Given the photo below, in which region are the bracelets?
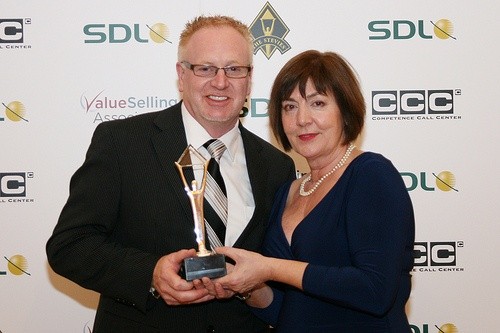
[233,289,254,301]
[149,286,162,299]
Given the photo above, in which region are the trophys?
[174,142,227,281]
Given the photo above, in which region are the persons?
[45,14,299,333]
[189,50,415,333]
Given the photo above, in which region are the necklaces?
[299,142,357,197]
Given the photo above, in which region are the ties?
[203,139,229,252]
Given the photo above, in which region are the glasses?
[182,59,253,78]
